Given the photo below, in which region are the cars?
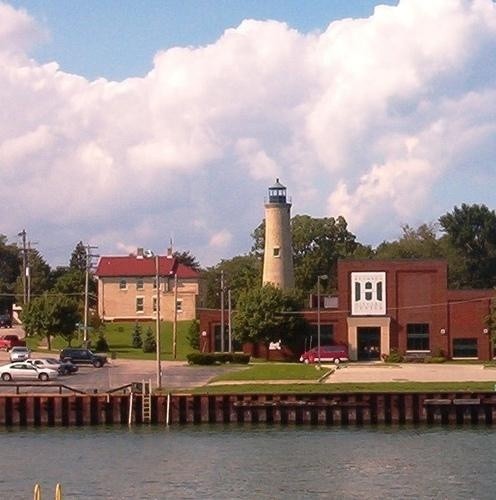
[0,333,81,383]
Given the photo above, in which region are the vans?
[298,345,350,364]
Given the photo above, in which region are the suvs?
[0,313,12,329]
[59,346,108,368]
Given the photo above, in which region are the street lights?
[26,240,40,305]
[170,270,177,359]
[144,248,163,390]
[222,284,233,354]
[17,227,26,304]
[316,274,329,370]
[215,287,225,353]
